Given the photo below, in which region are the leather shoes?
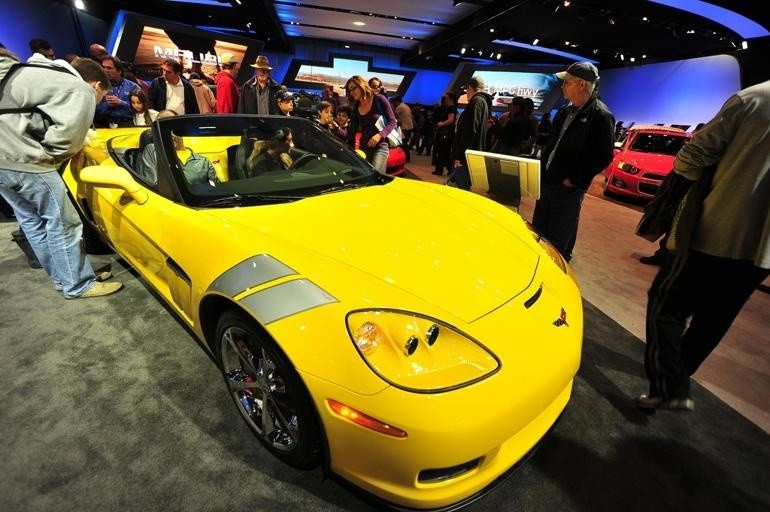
[637,388,694,413]
[640,253,668,266]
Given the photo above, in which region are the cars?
[604,125,695,205]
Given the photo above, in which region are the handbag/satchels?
[437,126,452,142]
[375,114,404,148]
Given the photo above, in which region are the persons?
[0,51,126,300]
[633,79,770,414]
[531,61,618,265]
[1,36,707,269]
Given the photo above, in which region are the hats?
[459,79,481,89]
[250,55,273,70]
[556,62,599,82]
[274,90,296,100]
[219,53,238,64]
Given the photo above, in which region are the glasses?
[370,84,382,88]
[349,86,357,92]
[564,79,582,85]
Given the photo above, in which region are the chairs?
[135,129,154,178]
[236,132,259,182]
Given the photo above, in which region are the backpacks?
[0,47,76,116]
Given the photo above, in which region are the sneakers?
[65,282,122,299]
[56,272,111,292]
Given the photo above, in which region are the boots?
[11,229,43,269]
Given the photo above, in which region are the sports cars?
[56,114,585,512]
